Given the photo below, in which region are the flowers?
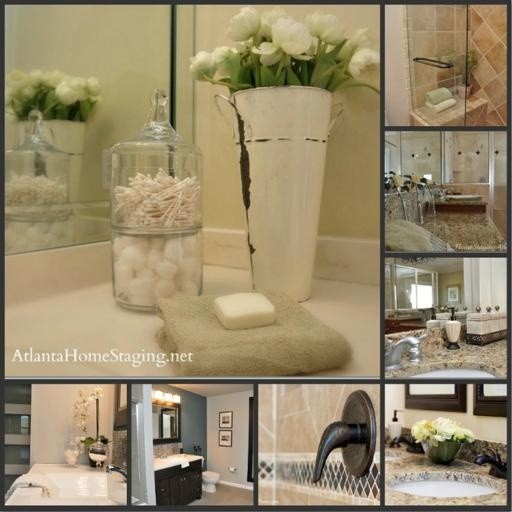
[190,6,380,88]
[384,171,410,193]
[73,386,112,449]
[412,418,477,447]
[402,173,427,195]
[2,68,101,124]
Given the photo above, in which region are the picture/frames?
[219,411,233,428]
[446,283,461,306]
[219,430,232,447]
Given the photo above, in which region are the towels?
[425,87,452,104]
[175,459,190,469]
[425,99,457,112]
[154,289,352,377]
[4,473,61,502]
[385,219,455,252]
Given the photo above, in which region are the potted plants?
[443,48,479,98]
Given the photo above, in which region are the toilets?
[200,470,221,494]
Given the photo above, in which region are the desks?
[425,200,488,214]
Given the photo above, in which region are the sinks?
[393,478,497,498]
[45,470,109,499]
[410,366,498,379]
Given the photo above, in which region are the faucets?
[472,448,507,480]
[389,435,425,455]
[386,335,421,371]
[105,462,127,483]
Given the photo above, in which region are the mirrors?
[0,1,199,259]
[405,384,467,412]
[152,403,181,445]
[113,383,127,431]
[473,384,508,417]
[385,258,474,336]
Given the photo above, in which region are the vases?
[88,446,108,468]
[215,88,345,303]
[26,118,86,203]
[421,439,463,465]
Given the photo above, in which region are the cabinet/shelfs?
[155,460,203,507]
